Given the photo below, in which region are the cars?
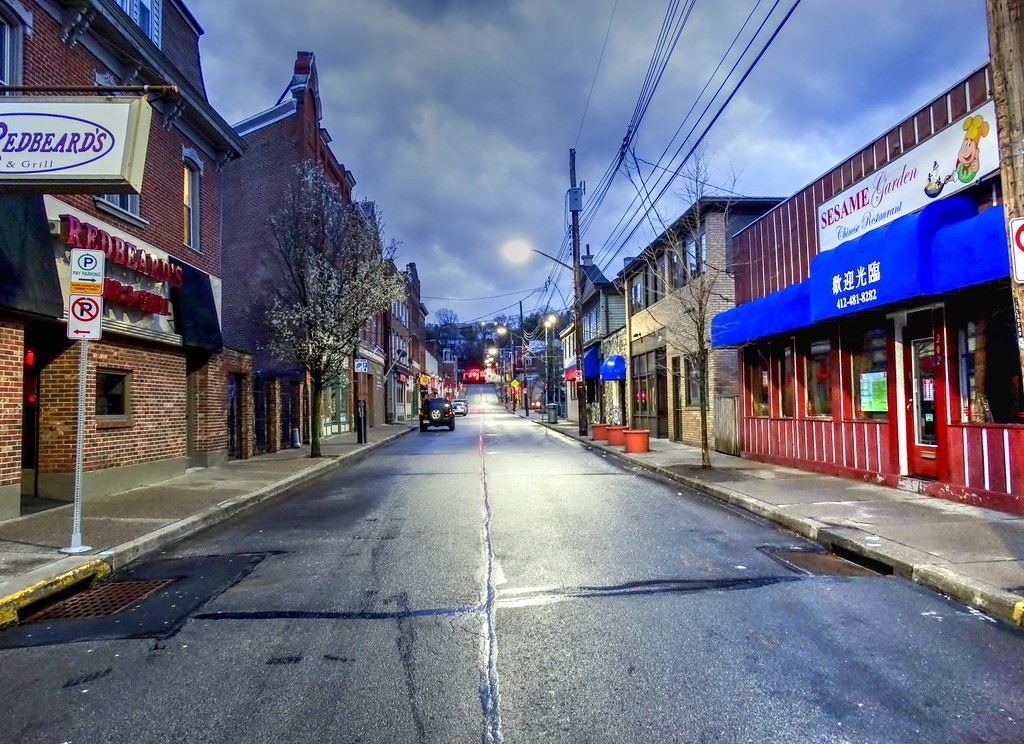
[532,395,564,410]
[451,402,467,416]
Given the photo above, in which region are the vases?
[622,429,650,454]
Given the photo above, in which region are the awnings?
[599,355,626,380]
[711,196,1010,350]
[564,347,600,381]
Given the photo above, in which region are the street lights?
[489,348,508,410]
[497,327,529,417]
[544,314,557,406]
[501,240,589,436]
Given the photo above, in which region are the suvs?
[419,397,457,432]
[455,399,469,412]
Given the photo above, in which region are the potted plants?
[586,383,630,446]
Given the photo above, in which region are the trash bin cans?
[548,403,558,423]
